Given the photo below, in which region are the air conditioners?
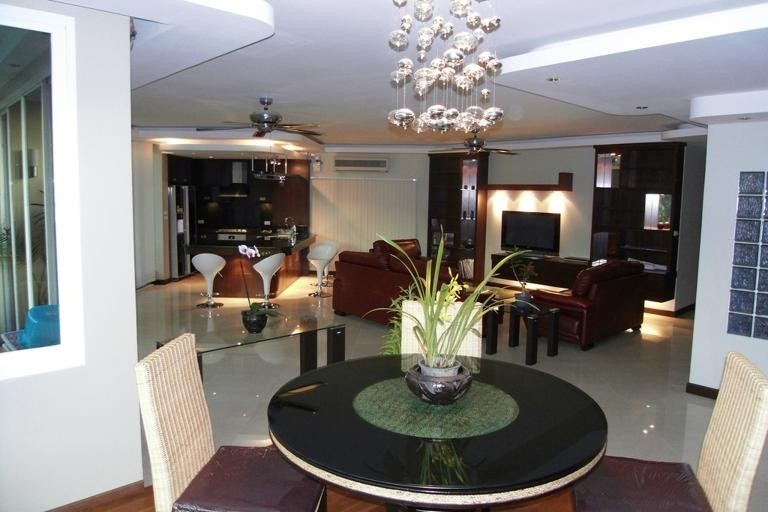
[332,156,390,174]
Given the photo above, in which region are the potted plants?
[240,300,286,334]
[357,224,544,403]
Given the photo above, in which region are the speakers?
[333,158,388,170]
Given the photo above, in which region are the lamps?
[385,1,504,136]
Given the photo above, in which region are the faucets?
[284,217,295,228]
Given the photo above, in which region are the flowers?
[505,244,539,294]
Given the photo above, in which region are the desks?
[483,296,560,365]
[155,311,346,386]
[266,347,611,510]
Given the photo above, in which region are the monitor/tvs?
[502,211,560,259]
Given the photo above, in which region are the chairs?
[303,238,340,297]
[192,252,228,309]
[568,349,768,511]
[400,299,483,361]
[252,250,287,308]
[136,329,330,511]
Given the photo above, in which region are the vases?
[514,291,532,308]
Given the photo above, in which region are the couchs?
[329,236,492,329]
[522,255,648,350]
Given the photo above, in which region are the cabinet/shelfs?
[589,141,687,302]
[426,152,490,286]
[491,250,592,288]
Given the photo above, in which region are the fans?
[194,96,327,145]
[428,131,519,158]
